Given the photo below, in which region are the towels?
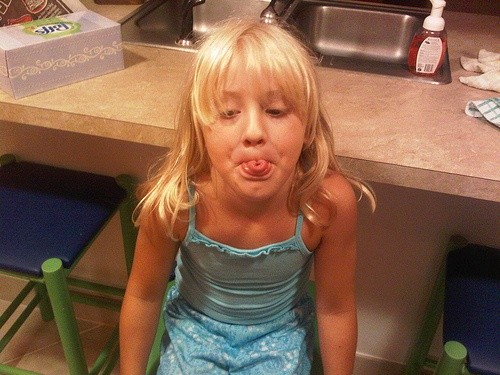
[458,48,500,93]
[464,97,500,128]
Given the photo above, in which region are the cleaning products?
[407,0,447,77]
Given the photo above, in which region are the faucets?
[176,0,205,47]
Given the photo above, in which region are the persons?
[117,15,377,375]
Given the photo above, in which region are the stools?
[0,153,142,375]
[145,278,324,375]
[401,234,500,375]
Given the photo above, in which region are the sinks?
[116,0,283,54]
[279,0,452,85]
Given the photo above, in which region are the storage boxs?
[0,9,124,100]
